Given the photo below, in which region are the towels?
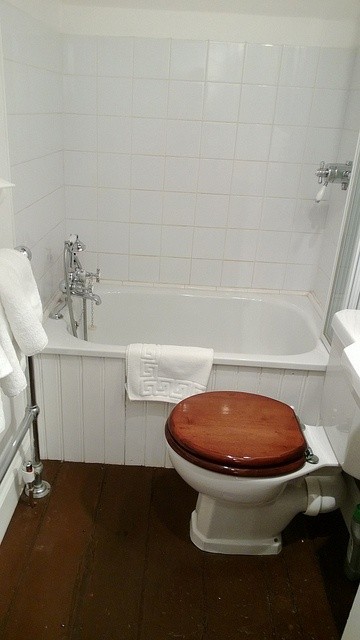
[0,247,48,397]
[125,342,213,405]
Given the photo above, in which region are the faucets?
[71,280,102,306]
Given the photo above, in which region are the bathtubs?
[28,276,344,471]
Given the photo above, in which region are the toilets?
[164,309,360,557]
